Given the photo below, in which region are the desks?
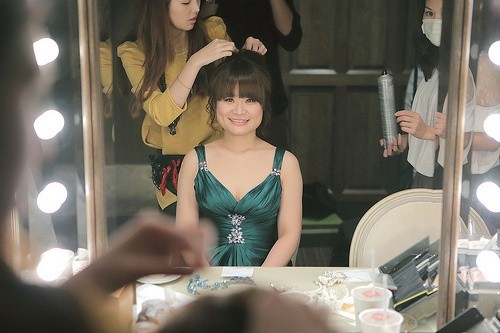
[130,267,387,333]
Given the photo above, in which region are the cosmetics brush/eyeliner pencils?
[413,248,440,280]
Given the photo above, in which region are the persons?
[118,0,268,219]
[39,0,115,254]
[190,0,309,151]
[379,0,499,242]
[0,0,116,333]
[172,48,303,267]
[155,286,353,333]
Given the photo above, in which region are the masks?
[421,19,442,48]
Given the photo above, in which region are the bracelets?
[206,1,217,4]
[176,76,191,89]
[176,77,193,91]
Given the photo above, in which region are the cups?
[73,257,90,275]
[352,286,392,333]
[359,309,403,333]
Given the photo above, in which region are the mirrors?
[77,0,473,333]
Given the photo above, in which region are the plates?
[132,284,195,322]
[137,274,181,284]
